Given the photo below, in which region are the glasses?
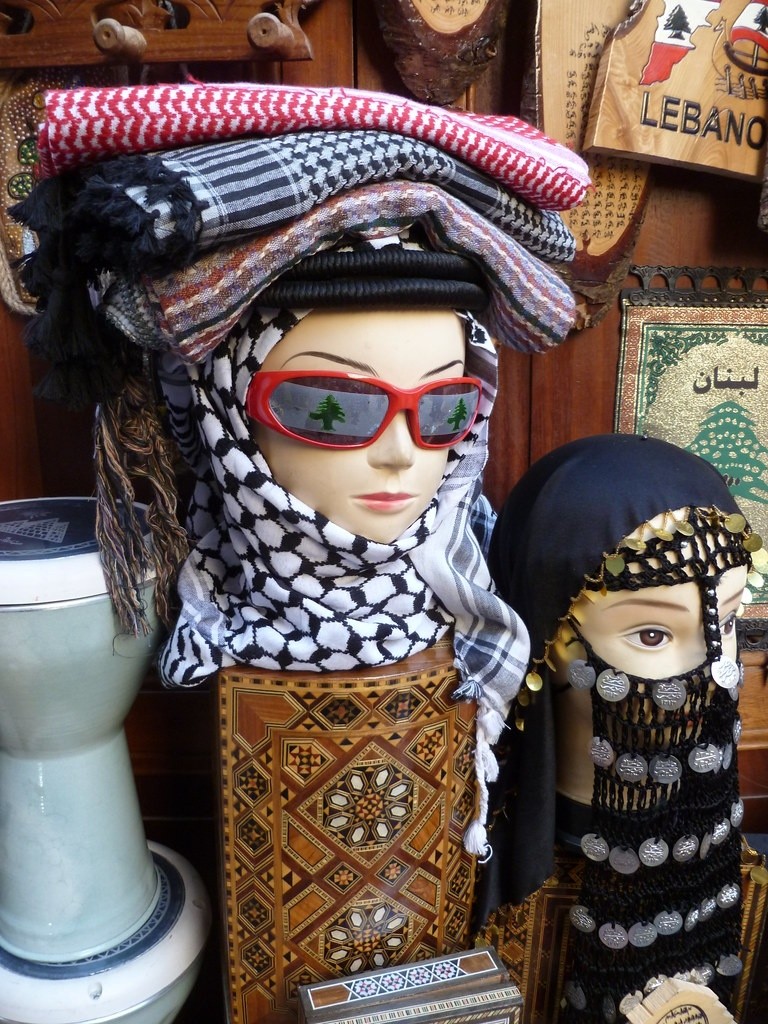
[246,370,483,451]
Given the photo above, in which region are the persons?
[160,222,493,670]
[470,434,768,812]
